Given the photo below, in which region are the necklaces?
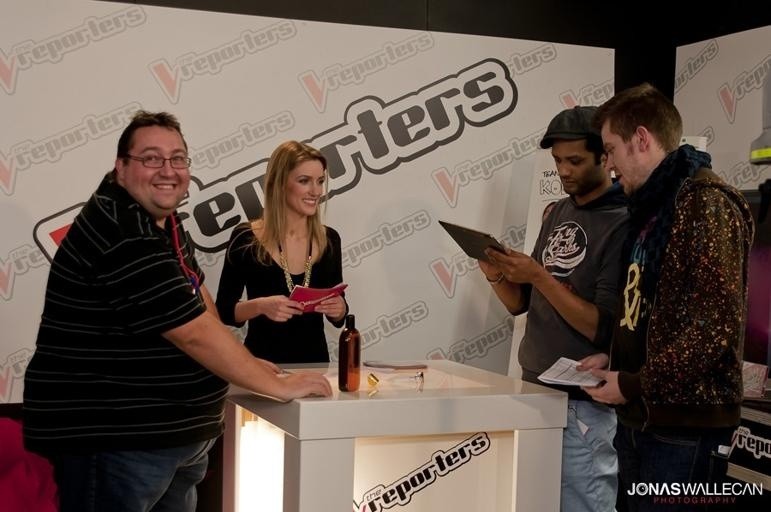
[275,234,313,293]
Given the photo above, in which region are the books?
[289,282,348,313]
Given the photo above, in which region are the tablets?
[438,219,510,261]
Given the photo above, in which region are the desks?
[220,358,570,511]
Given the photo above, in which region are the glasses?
[124,154,192,169]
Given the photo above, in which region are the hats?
[539,106,600,148]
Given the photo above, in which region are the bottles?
[338,314,362,392]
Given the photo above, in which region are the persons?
[479,106,630,510]
[215,141,349,367]
[23,113,331,512]
[573,83,755,511]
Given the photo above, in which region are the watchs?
[487,275,505,285]
